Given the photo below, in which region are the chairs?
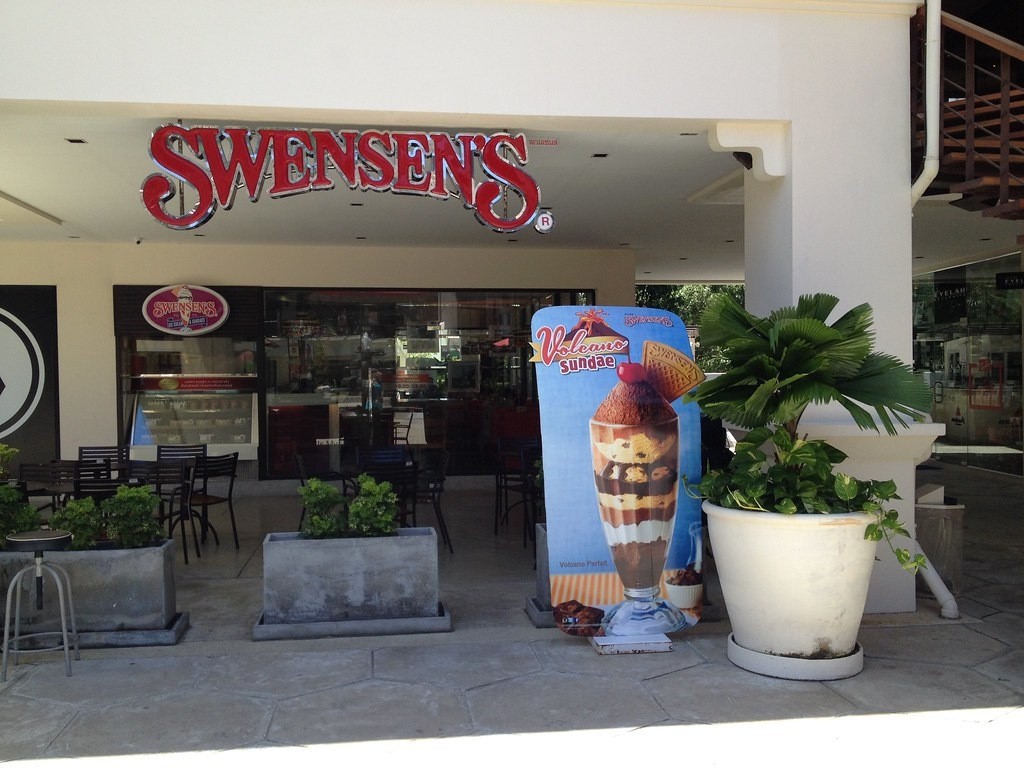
[19,445,239,565]
[494,436,546,571]
[293,411,454,554]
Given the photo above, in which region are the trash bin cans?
[916,502,966,602]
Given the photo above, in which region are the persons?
[365,372,384,411]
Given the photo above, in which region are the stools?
[1,531,80,682]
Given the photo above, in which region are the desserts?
[591,378,678,589]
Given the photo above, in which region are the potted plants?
[0,443,190,649]
[252,472,452,642]
[684,293,934,680]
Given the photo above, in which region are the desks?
[45,478,147,494]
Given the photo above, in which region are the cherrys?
[617,345,644,383]
